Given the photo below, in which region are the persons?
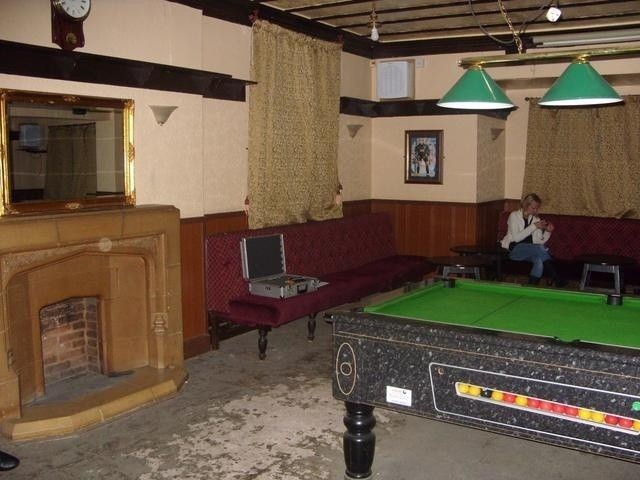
[0,450,21,474]
[501,192,557,286]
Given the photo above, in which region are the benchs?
[205,211,436,361]
[496,210,640,274]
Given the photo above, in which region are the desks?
[330,272,640,479]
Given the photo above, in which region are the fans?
[377,61,416,100]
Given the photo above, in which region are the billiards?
[458,383,640,432]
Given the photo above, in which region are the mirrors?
[0,88,134,217]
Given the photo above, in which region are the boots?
[543,259,568,288]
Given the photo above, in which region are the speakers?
[19,124,42,147]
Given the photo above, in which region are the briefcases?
[240,234,317,297]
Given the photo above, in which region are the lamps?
[435,46,639,110]
[370,2,380,42]
[150,105,177,126]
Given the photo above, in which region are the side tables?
[576,255,635,296]
[425,245,507,280]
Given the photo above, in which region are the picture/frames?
[405,131,442,184]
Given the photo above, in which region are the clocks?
[50,0,91,51]
